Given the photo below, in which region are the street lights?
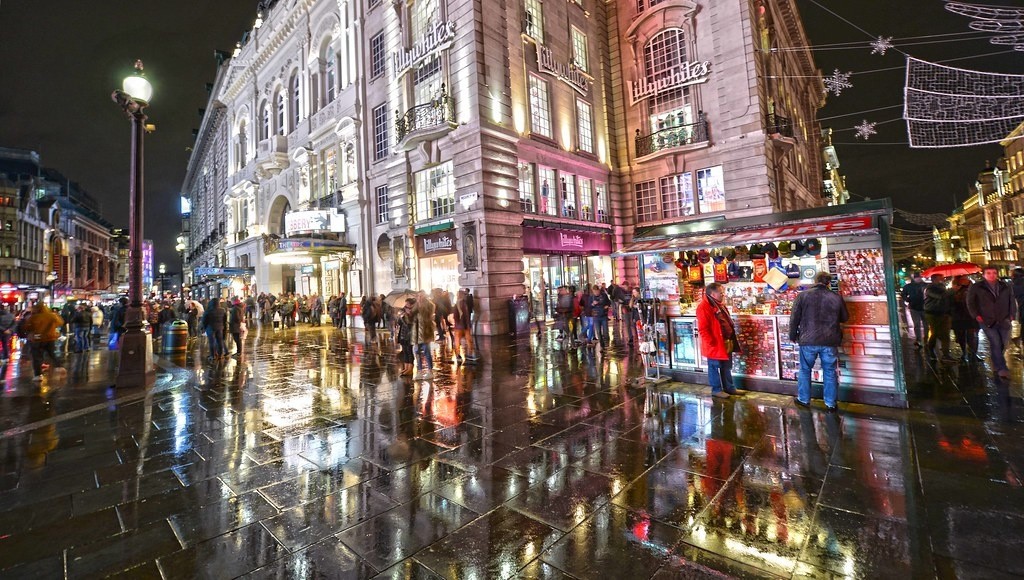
[45,275,55,296]
[110,57,156,389]
[159,263,166,301]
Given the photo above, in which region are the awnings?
[609,214,881,261]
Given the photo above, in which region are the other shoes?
[412,373,423,380]
[32,375,43,381]
[557,336,563,340]
[224,352,229,356]
[794,398,810,408]
[403,370,412,375]
[423,373,433,379]
[713,391,730,399]
[467,355,475,360]
[930,351,936,360]
[401,369,407,374]
[827,405,838,414]
[457,355,461,361]
[730,390,746,395]
[998,369,1009,376]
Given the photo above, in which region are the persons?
[901,271,929,349]
[506,279,640,350]
[788,273,850,416]
[925,266,1024,364]
[696,283,746,399]
[358,289,480,381]
[966,267,1016,377]
[0,285,348,394]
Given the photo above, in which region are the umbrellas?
[919,263,983,278]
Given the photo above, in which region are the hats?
[914,273,919,278]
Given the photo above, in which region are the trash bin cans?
[507,295,531,336]
